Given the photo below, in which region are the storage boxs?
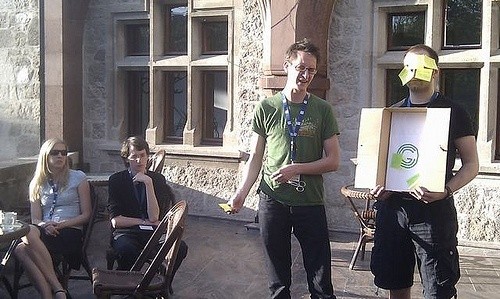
[354,107,451,192]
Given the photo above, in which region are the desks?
[341,182,379,270]
[84,172,110,187]
[0,213,30,299]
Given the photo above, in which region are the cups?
[0,209,18,231]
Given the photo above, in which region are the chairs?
[92,199,190,299]
[106,183,176,270]
[12,191,98,299]
[147,148,166,176]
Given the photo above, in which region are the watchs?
[445,186,453,200]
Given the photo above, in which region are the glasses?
[289,60,318,76]
[49,150,67,156]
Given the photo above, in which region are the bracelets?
[142,218,146,225]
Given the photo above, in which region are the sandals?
[54,289,71,299]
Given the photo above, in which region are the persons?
[107,136,188,287]
[225,39,341,299]
[14,136,92,299]
[371,44,480,299]
